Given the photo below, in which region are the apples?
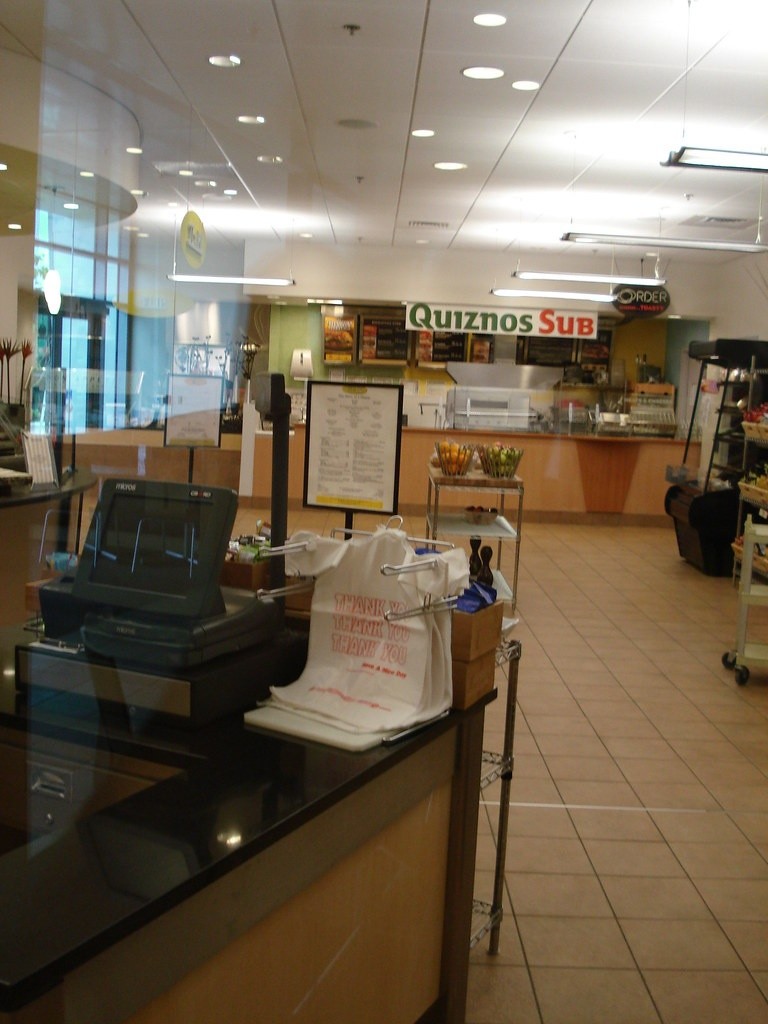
[478,442,519,476]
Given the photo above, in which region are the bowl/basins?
[463,510,499,525]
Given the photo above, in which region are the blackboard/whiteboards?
[359,316,412,365]
[577,327,613,385]
[516,334,578,366]
[415,329,471,368]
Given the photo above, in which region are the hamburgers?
[324,331,352,350]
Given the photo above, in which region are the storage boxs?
[450,648,497,709]
[451,600,505,660]
[221,560,314,611]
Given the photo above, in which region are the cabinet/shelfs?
[666,339,768,686]
[424,462,525,613]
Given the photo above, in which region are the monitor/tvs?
[70,477,238,617]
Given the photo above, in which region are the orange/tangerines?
[438,441,470,474]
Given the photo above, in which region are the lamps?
[658,0,768,174]
[166,208,296,287]
[487,156,768,303]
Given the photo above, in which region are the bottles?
[639,354,648,382]
[635,354,642,379]
